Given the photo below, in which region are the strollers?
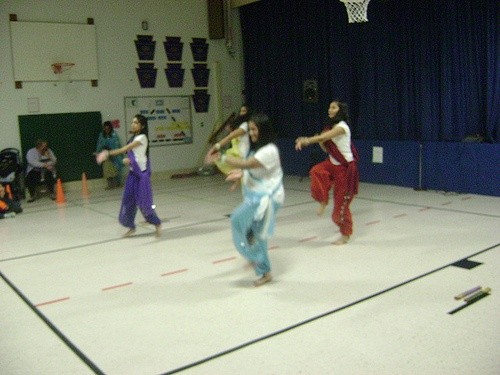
[0,148,25,214]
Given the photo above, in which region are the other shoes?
[27,192,41,202]
[48,190,56,200]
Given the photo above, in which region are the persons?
[24,138,57,203]
[97,114,162,237]
[295,100,359,245]
[95,121,123,190]
[206,102,254,192]
[215,113,285,285]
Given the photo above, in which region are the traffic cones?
[54,179,66,204]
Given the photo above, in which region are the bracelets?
[304,137,308,146]
[106,150,111,158]
[215,142,221,151]
[221,154,228,164]
